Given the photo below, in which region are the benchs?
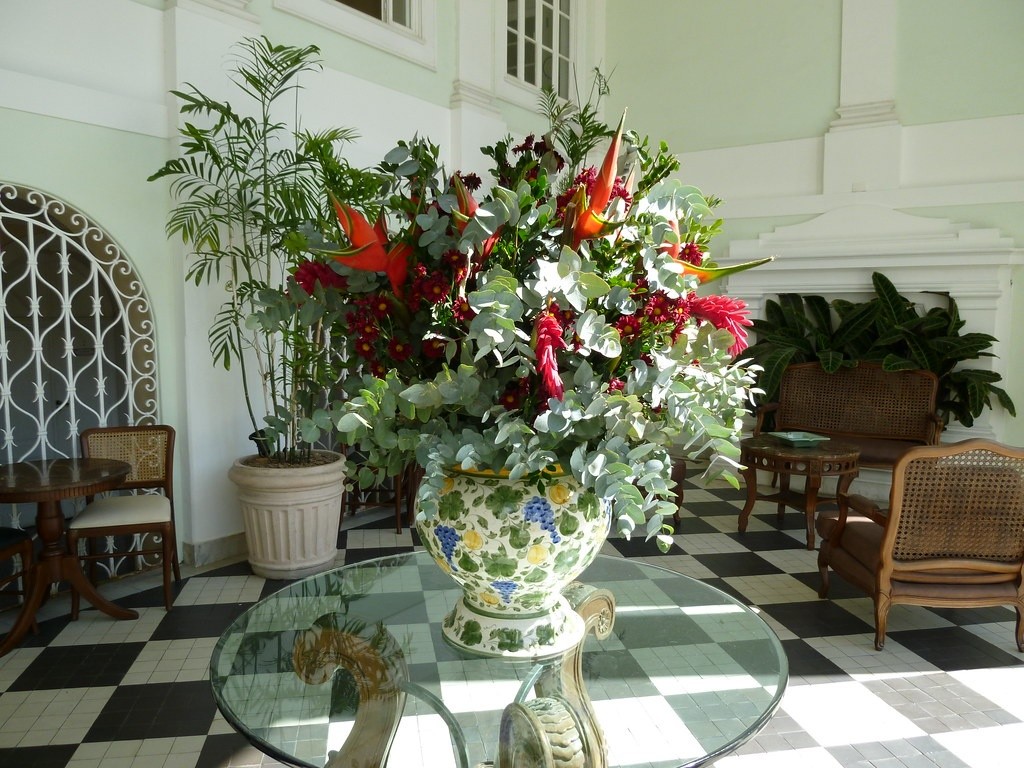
[754,361,944,487]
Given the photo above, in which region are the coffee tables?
[737,434,862,551]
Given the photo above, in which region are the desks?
[0,458,139,657]
[209,551,789,768]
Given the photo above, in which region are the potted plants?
[145,36,405,580]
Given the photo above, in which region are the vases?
[413,465,615,660]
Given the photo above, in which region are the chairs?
[66,425,181,621]
[816,438,1024,652]
[340,441,415,534]
[0,527,38,634]
[632,458,686,531]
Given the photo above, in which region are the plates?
[767,431,831,448]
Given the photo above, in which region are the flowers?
[246,53,766,537]
